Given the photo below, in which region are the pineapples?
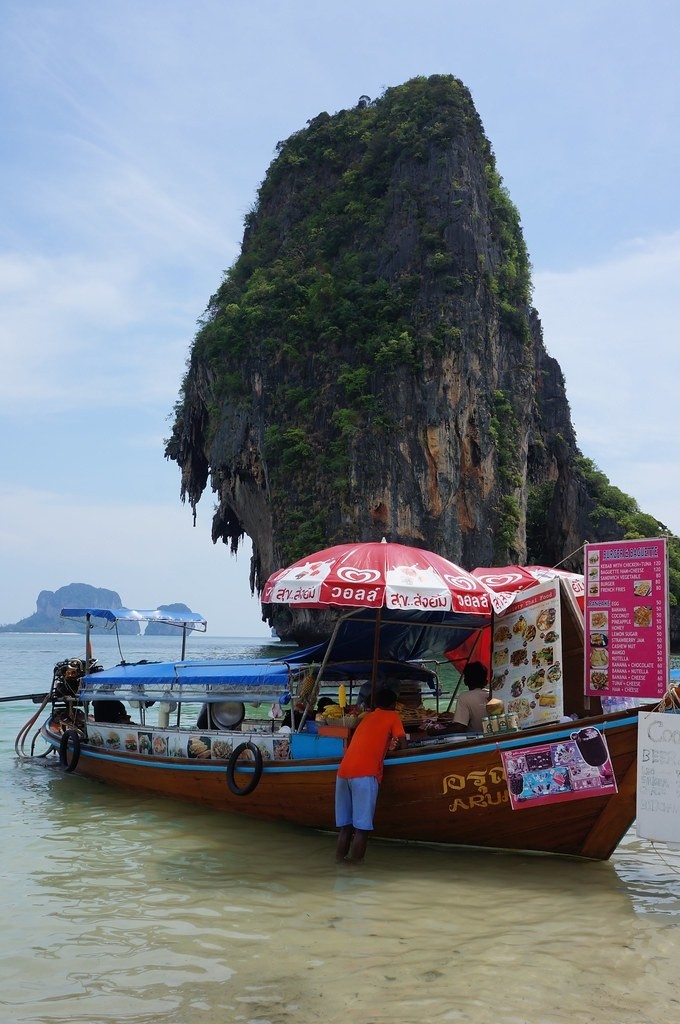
[302,666,315,694]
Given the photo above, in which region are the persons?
[92,683,137,725]
[196,683,223,730]
[426,660,491,739]
[278,679,374,734]
[335,689,410,859]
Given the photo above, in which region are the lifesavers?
[59,730,80,772]
[227,741,263,796]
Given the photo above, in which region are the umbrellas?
[441,562,585,684]
[261,537,516,720]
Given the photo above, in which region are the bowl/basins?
[535,609,555,632]
[526,675,544,692]
[546,665,561,684]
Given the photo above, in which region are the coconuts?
[486,698,504,716]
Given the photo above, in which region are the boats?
[38,657,680,860]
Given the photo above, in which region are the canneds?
[480,711,518,733]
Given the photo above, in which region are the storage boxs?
[305,721,327,734]
[318,725,351,738]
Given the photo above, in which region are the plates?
[506,696,534,720]
[590,633,608,647]
[494,624,508,645]
[589,611,608,631]
[512,621,527,638]
[590,648,609,670]
[522,625,536,643]
[530,646,553,667]
[510,648,528,668]
[509,677,525,698]
[492,650,510,670]
[543,629,560,646]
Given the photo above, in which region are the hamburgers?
[106,732,120,749]
[125,733,137,752]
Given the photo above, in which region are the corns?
[322,705,342,719]
[358,712,368,718]
[190,743,211,759]
[339,684,346,707]
